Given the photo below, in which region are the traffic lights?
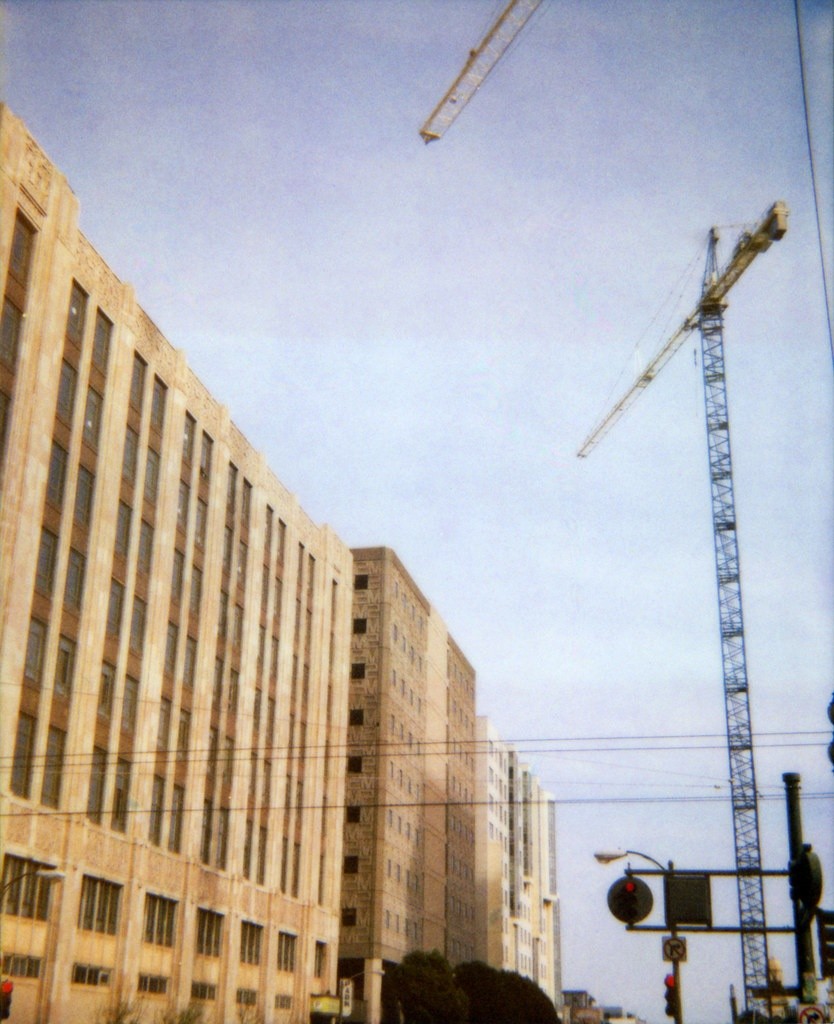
[664,974,677,1017]
[622,878,635,924]
[818,909,834,978]
[1,980,15,1017]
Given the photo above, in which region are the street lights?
[340,969,384,1024]
[591,847,682,1024]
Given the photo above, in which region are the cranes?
[573,194,794,1023]
